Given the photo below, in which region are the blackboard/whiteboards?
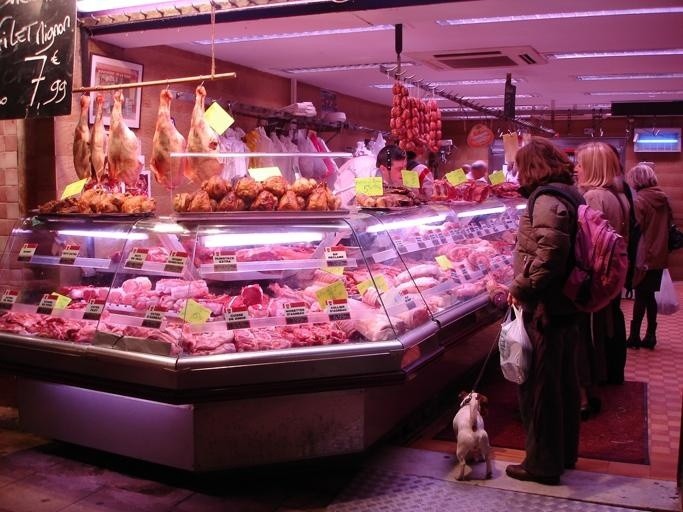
[0,0,77,120]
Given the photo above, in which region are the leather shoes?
[507,464,561,485]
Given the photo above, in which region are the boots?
[626,320,642,350]
[642,319,657,350]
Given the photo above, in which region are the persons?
[577,141,673,419]
[493,135,587,486]
[335,145,488,208]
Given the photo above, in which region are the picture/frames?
[89,55,144,132]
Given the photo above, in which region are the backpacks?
[529,185,630,313]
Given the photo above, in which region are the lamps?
[636,139,680,145]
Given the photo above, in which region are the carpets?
[432,372,650,464]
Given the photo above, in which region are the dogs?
[452,391,492,480]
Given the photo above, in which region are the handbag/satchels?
[668,225,683,250]
